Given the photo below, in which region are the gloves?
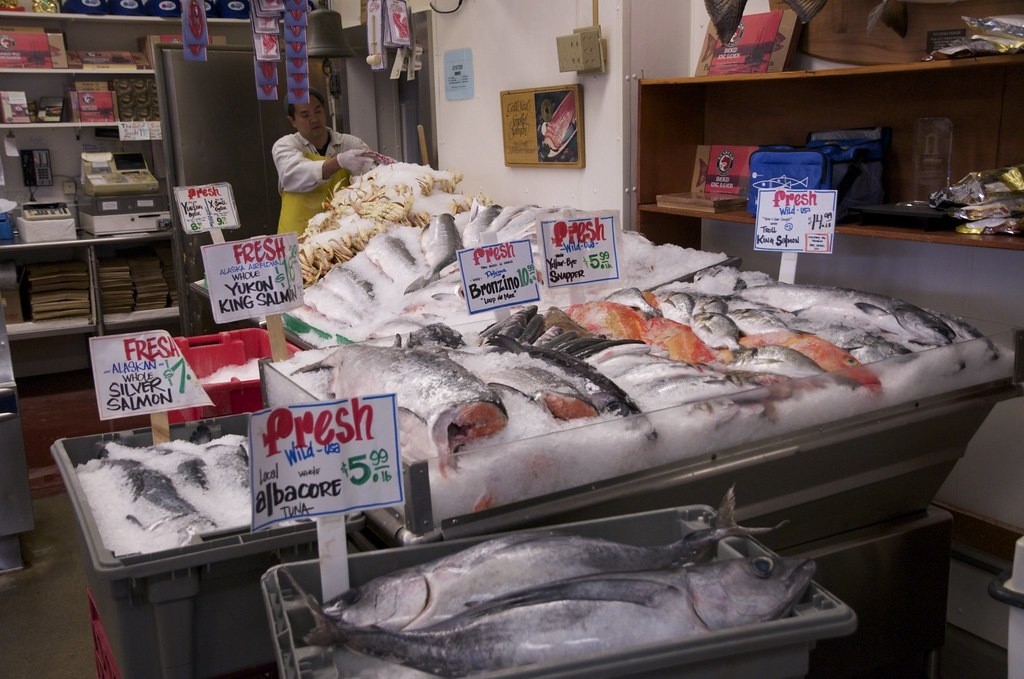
[337,149,373,170]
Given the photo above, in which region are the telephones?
[19,149,53,187]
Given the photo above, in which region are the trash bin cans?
[988,536,1024,679]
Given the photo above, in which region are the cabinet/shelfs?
[636,55,1024,255]
[0,19,259,337]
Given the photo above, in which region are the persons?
[272,88,378,239]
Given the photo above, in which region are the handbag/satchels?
[749,126,891,223]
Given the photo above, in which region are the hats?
[283,87,325,113]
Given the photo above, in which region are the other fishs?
[289,265,1001,510]
[83,433,250,544]
[278,554,821,679]
[297,164,495,289]
[293,205,584,339]
[322,487,790,631]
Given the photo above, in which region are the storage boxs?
[51,414,366,678]
[0,26,153,70]
[0,79,120,124]
[259,503,859,678]
[0,266,28,323]
[167,329,302,424]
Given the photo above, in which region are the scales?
[20,201,72,221]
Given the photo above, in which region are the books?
[30,248,180,322]
